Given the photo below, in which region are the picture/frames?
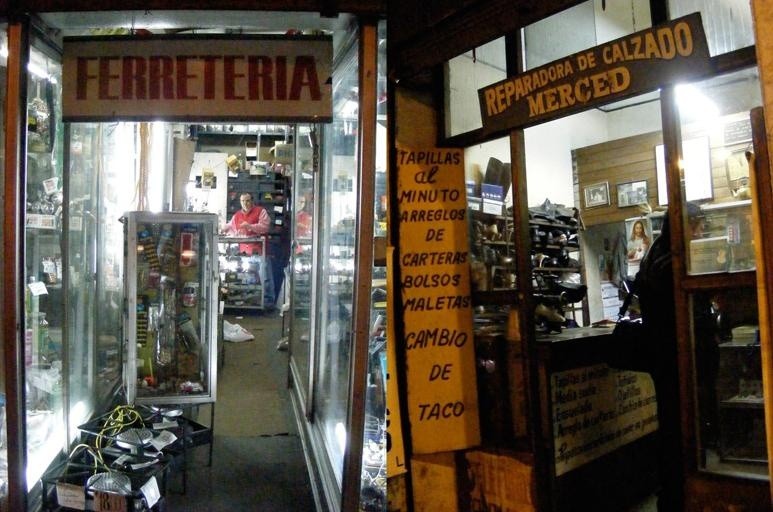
[615,179,649,208]
[583,180,611,210]
[723,117,753,147]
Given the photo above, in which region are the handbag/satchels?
[608,320,652,372]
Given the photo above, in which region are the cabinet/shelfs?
[218,169,287,316]
[40,405,186,511]
[470,209,590,328]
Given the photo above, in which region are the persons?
[628,187,646,204]
[296,191,312,237]
[221,191,276,316]
[636,200,721,512]
[627,221,649,261]
